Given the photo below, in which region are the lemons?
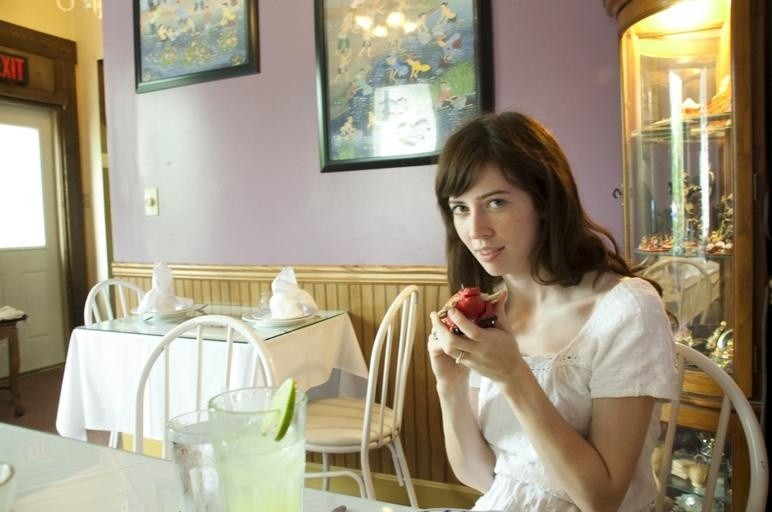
[260,378,296,443]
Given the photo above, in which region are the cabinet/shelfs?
[598,0,772,512]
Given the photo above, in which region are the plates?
[129,296,194,320]
[241,305,314,327]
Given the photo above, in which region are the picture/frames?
[314,0,496,173]
[132,0,262,94]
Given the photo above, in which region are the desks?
[0,305,31,420]
[75,305,347,483]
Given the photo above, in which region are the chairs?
[284,284,422,511]
[83,279,182,455]
[135,314,280,511]
[640,339,769,512]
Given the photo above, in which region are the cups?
[168,408,224,511]
[209,385,307,511]
[687,465,707,488]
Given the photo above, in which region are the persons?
[332,3,456,138]
[148,0,238,42]
[427,112,679,512]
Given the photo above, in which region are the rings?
[454,351,465,364]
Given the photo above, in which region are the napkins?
[270,265,318,320]
[135,260,182,314]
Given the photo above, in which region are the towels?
[266,262,320,322]
[127,259,186,319]
